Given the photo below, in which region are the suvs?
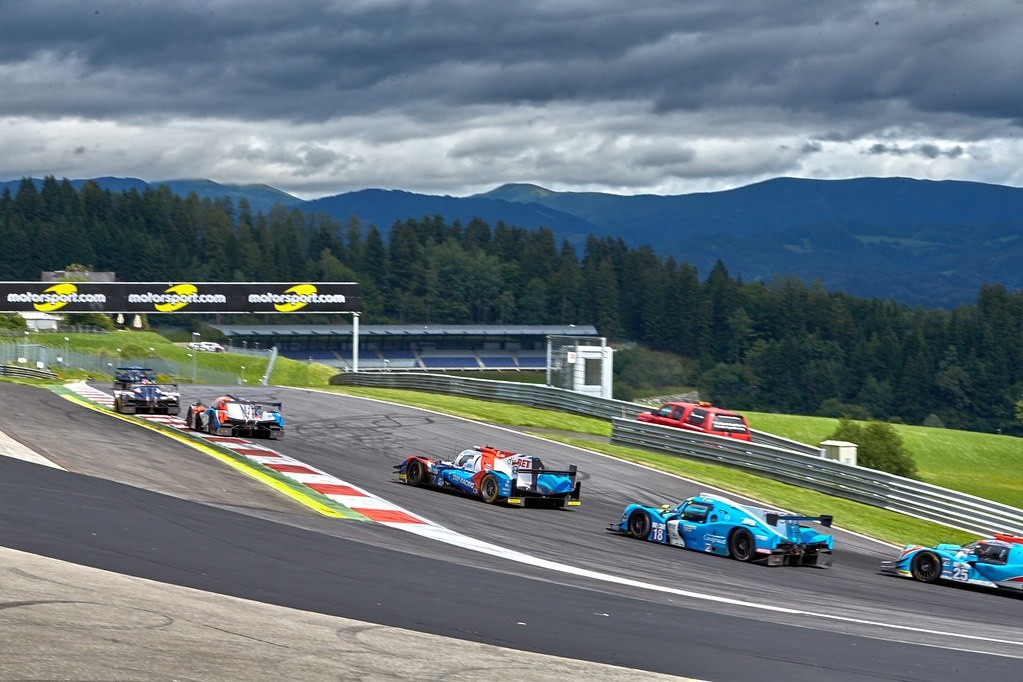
[637,401,752,442]
